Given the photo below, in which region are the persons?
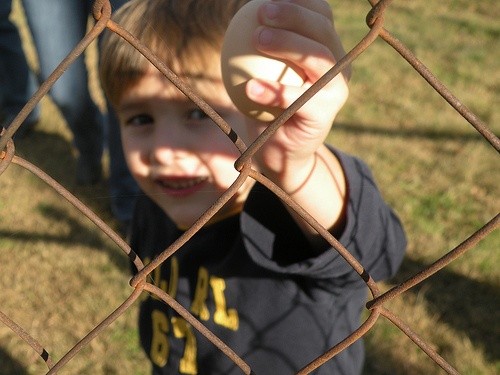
[20,0,151,220]
[0,0,42,140]
[97,0,408,375]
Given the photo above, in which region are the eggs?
[219,0,308,122]
